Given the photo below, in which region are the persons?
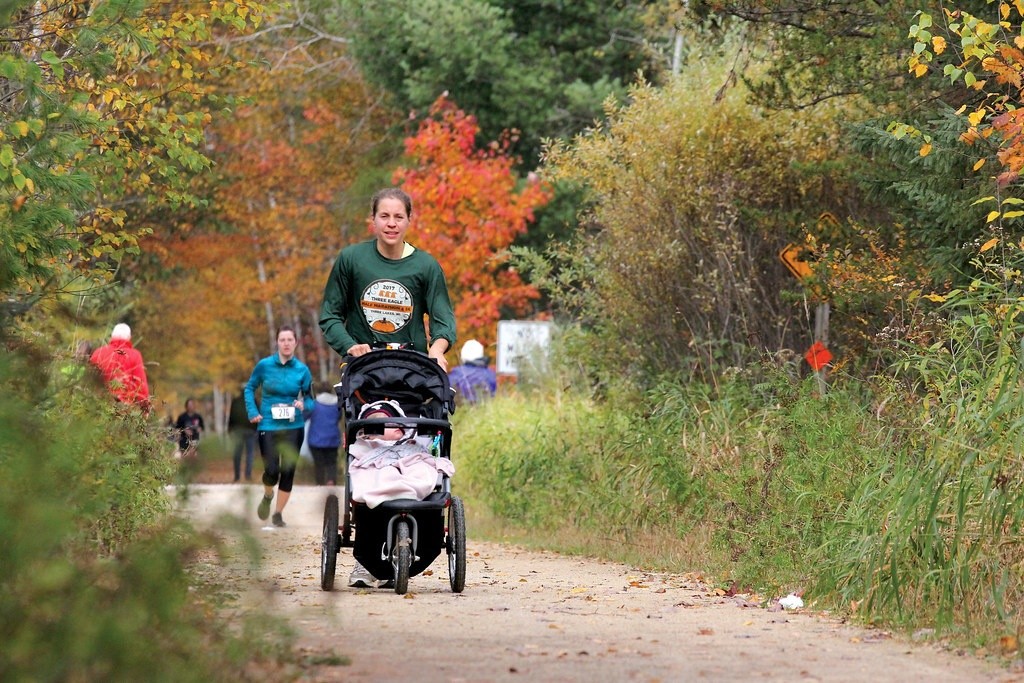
[85,323,153,425]
[319,190,457,588]
[446,339,496,405]
[228,383,260,484]
[244,326,316,526]
[174,397,205,503]
[348,401,439,509]
[302,382,341,486]
[50,337,97,395]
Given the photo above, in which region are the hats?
[461,340,483,363]
[111,323,131,340]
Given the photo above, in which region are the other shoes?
[272,512,285,527]
[258,490,274,520]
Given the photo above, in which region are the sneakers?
[347,560,374,587]
[377,579,395,588]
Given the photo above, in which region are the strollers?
[318,347,466,594]
